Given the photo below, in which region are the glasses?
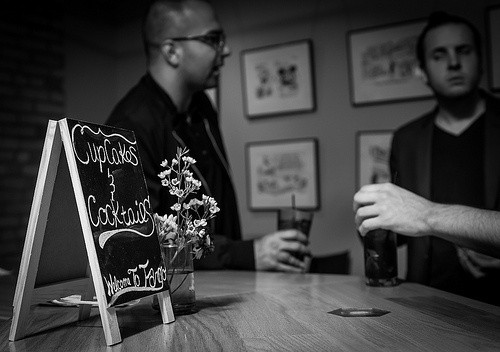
[168,29,226,49]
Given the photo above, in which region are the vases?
[160,243,199,315]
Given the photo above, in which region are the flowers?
[153,148,220,272]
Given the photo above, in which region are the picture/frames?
[483,4,500,93]
[239,37,317,120]
[245,137,322,213]
[354,129,393,193]
[346,17,436,108]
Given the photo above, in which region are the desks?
[0,269,500,352]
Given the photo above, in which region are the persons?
[389,14,500,308]
[103,0,310,274]
[353,182,500,258]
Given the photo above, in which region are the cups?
[361,204,398,287]
[161,242,196,316]
[278,207,314,268]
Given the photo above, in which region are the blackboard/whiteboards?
[11,117,170,310]
